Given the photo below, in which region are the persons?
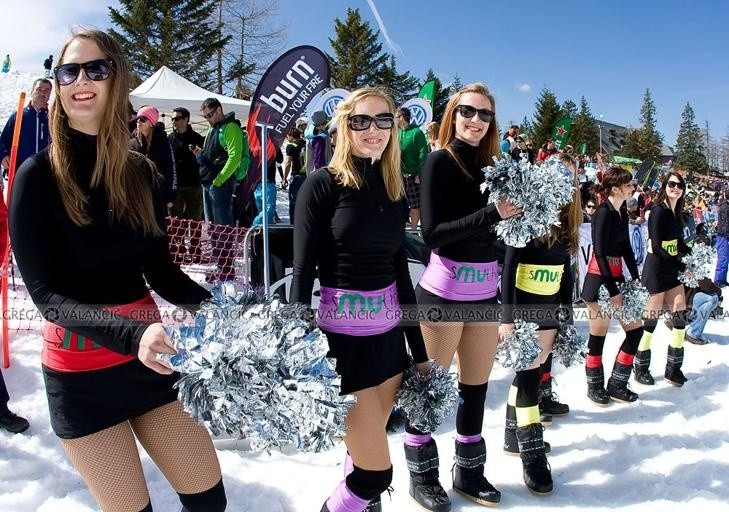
[1,28,729,511]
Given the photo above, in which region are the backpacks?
[289,139,307,175]
[218,123,251,181]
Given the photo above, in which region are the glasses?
[455,103,495,123]
[202,110,217,120]
[667,181,686,190]
[345,113,394,132]
[54,58,117,85]
[136,118,148,124]
[586,205,597,210]
[171,115,184,123]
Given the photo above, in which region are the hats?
[133,106,159,128]
[310,110,327,125]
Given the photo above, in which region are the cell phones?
[188,144,196,150]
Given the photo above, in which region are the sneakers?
[685,330,709,346]
[1,410,30,434]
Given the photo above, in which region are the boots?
[606,358,639,403]
[453,438,503,501]
[517,423,554,497]
[584,364,609,404]
[503,403,552,455]
[538,369,571,417]
[664,345,688,386]
[633,348,655,385]
[402,441,452,510]
[319,492,384,511]
